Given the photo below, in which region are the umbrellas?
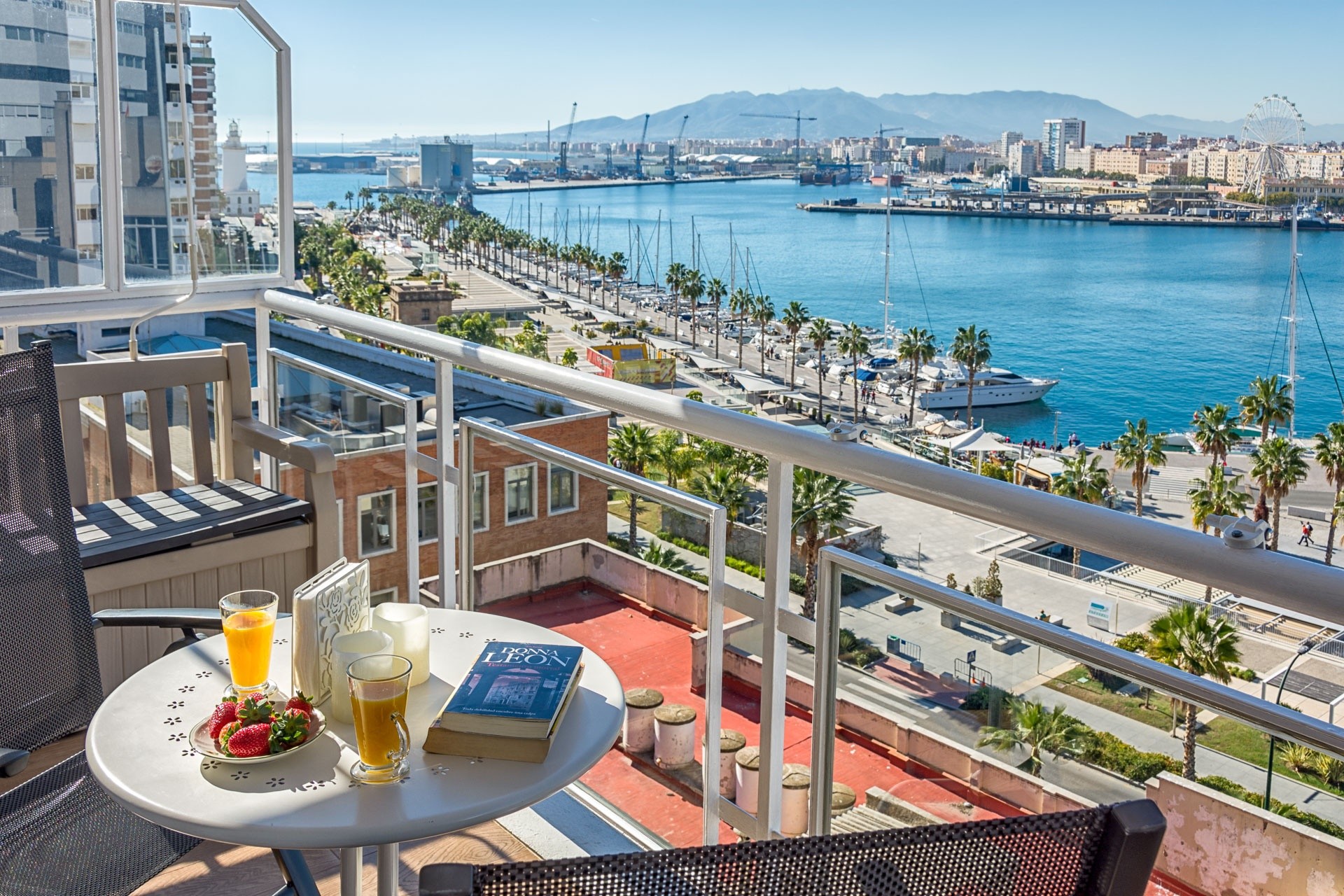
[879,415,904,443]
[916,413,967,432]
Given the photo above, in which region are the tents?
[916,428,1013,477]
[923,422,965,439]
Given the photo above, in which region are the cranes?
[635,114,650,180]
[664,114,690,176]
[873,123,904,164]
[738,108,818,173]
[559,102,578,180]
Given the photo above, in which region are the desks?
[85,607,626,896]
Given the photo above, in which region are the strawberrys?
[235,692,270,725]
[285,690,314,718]
[276,707,311,746]
[240,714,279,727]
[227,722,282,757]
[210,696,239,739]
[218,720,246,754]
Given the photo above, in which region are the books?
[423,640,585,763]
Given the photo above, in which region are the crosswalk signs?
[966,650,976,664]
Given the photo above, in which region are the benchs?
[1287,505,1325,522]
[0,343,338,745]
[1049,615,1064,625]
[991,636,1020,651]
[1222,467,1232,475]
[885,598,914,612]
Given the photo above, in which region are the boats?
[1154,422,1277,448]
[895,351,1062,410]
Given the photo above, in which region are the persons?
[1023,438,1046,449]
[1068,432,1076,447]
[690,324,693,333]
[862,406,867,416]
[900,413,909,427]
[1050,442,1063,451]
[1101,483,1117,498]
[1101,442,1112,450]
[954,411,959,421]
[999,435,1011,443]
[1304,521,1314,544]
[1297,524,1309,547]
[860,389,876,404]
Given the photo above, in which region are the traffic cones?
[981,677,986,687]
[970,673,977,683]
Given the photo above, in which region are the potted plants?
[796,402,831,426]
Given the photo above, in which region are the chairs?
[0,341,320,896]
[416,799,1168,896]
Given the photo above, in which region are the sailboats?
[498,179,989,396]
[1182,206,1339,457]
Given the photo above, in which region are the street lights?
[757,503,768,580]
[1265,639,1317,811]
[1054,411,1062,461]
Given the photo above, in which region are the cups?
[345,654,413,784]
[330,630,394,724]
[218,589,279,701]
[373,602,430,688]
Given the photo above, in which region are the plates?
[189,700,327,765]
